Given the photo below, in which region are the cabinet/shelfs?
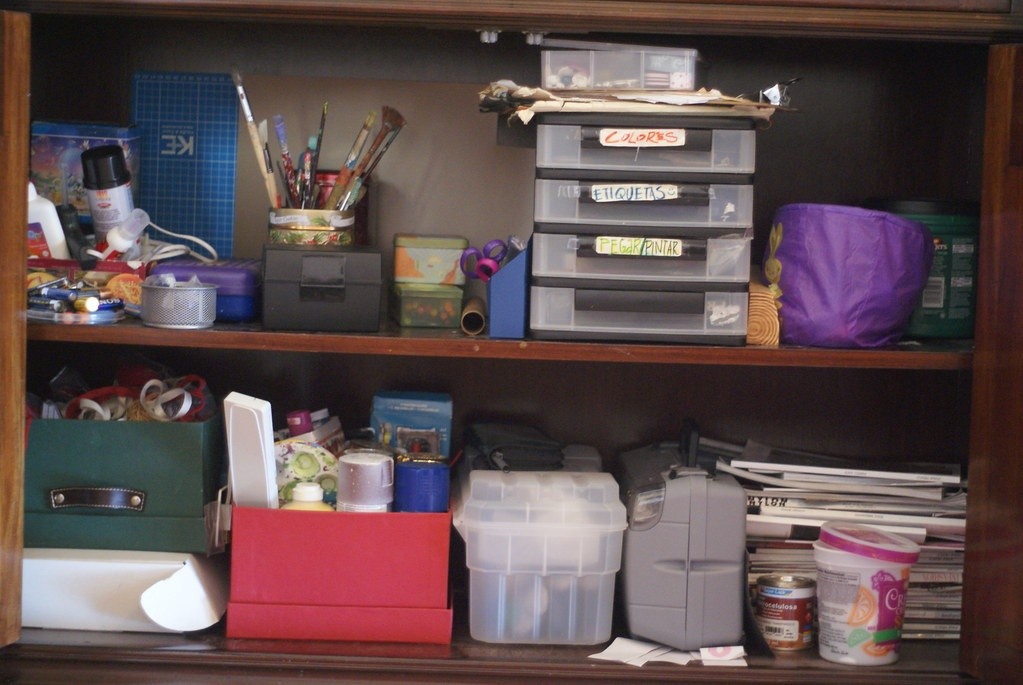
[0,0,1023,684]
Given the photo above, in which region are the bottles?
[814,514,922,667]
[28,180,70,262]
[81,144,150,261]
[895,193,982,351]
[285,407,315,439]
[277,480,336,511]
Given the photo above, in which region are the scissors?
[459,239,508,283]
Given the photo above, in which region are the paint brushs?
[227,63,409,212]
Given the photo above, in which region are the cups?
[744,567,818,662]
[278,164,372,205]
[266,205,358,252]
[336,452,451,513]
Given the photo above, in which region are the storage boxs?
[151,260,259,322]
[616,419,747,652]
[531,114,757,346]
[29,118,141,225]
[370,389,452,455]
[540,36,697,93]
[394,234,469,286]
[24,416,223,555]
[259,243,384,333]
[388,281,463,328]
[275,416,345,457]
[451,444,631,646]
[226,500,455,646]
[22,546,228,634]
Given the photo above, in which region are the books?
[697,436,967,639]
